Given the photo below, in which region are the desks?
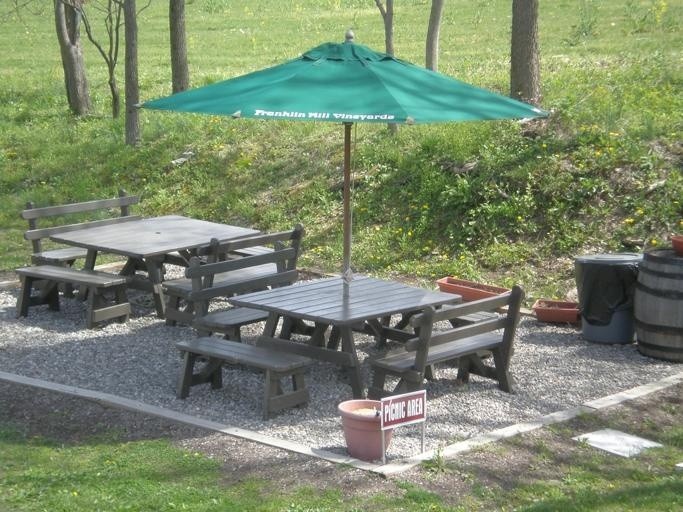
[49,215,261,317]
[228,275,462,399]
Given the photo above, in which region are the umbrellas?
[131,27,550,297]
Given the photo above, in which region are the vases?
[436,277,508,302]
[532,299,580,323]
[338,400,393,460]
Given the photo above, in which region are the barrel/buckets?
[632,247,679,363]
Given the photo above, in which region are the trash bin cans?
[573,252,643,345]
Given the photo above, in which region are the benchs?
[162,222,307,326]
[368,284,523,401]
[184,241,298,371]
[20,189,142,298]
[228,246,275,257]
[443,304,498,328]
[17,265,128,326]
[177,337,312,422]
